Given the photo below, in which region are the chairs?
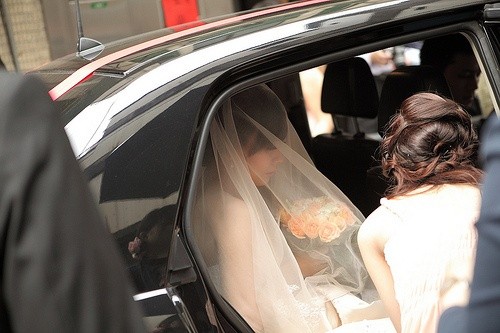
[311,57,386,218]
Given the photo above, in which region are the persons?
[191,83,388,333]
[0,57,147,333]
[358,92,500,333]
[355,45,396,75]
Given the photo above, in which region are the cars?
[0,0,500,333]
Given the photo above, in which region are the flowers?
[278,195,356,244]
